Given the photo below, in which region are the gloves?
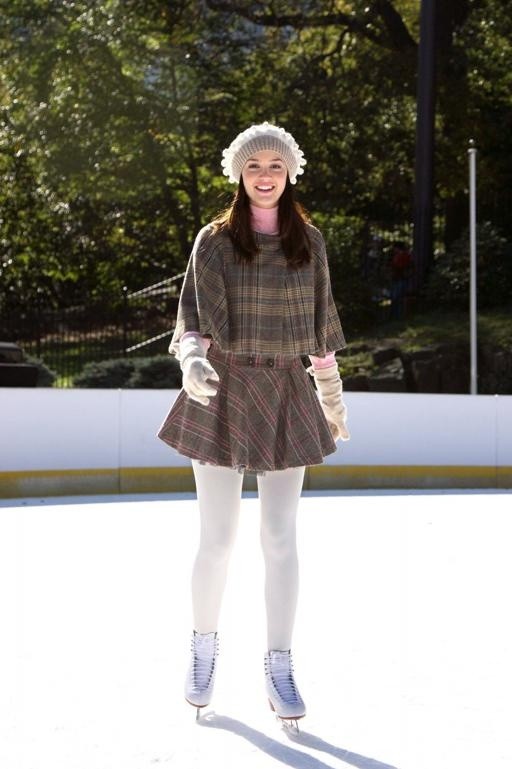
[307,364,349,444]
[176,336,220,406]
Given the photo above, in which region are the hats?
[222,124,307,183]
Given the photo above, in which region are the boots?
[186,630,220,710]
[263,648,306,720]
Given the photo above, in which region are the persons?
[156,119,350,721]
[392,242,414,319]
